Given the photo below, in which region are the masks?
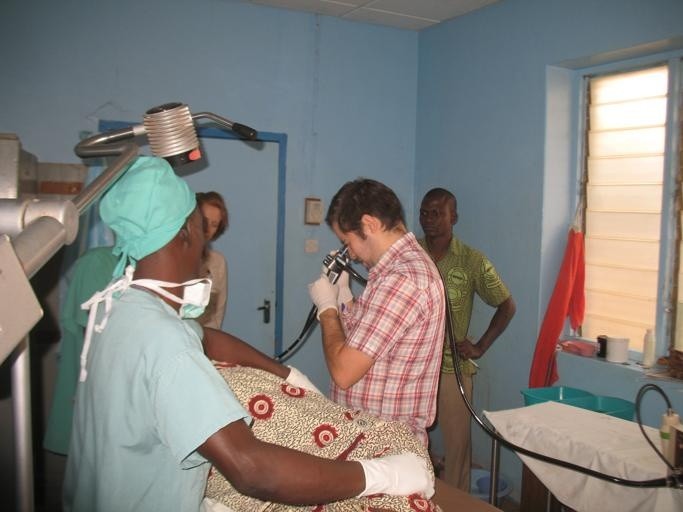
[131,277,213,319]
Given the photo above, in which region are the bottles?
[659,412,682,453]
[641,328,655,369]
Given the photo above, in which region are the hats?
[98,154,198,278]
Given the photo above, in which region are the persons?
[64,156,435,511]
[193,192,228,332]
[306,180,445,451]
[413,189,517,496]
[43,247,134,456]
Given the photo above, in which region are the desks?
[482,399,683,512]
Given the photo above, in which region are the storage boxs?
[520,386,594,406]
[556,396,637,421]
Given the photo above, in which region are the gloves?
[305,271,339,321]
[282,363,326,396]
[351,452,435,500]
[320,250,354,306]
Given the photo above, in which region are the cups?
[604,335,630,365]
[596,334,607,357]
